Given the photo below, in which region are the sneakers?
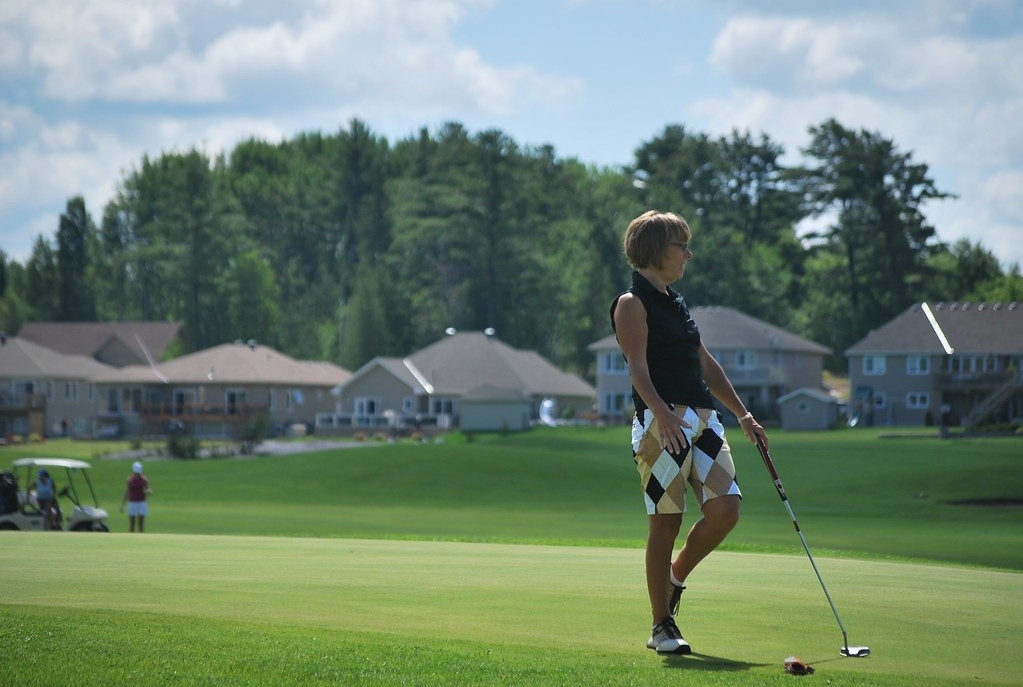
[647,616,691,654]
[668,562,686,616]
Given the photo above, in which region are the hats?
[37,470,46,477]
[132,462,143,473]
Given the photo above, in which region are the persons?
[610,210,770,654]
[62,416,67,436]
[119,461,152,533]
[30,469,62,530]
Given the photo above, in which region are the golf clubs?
[753,432,871,657]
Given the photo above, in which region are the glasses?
[668,242,688,252]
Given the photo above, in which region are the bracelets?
[738,412,751,423]
